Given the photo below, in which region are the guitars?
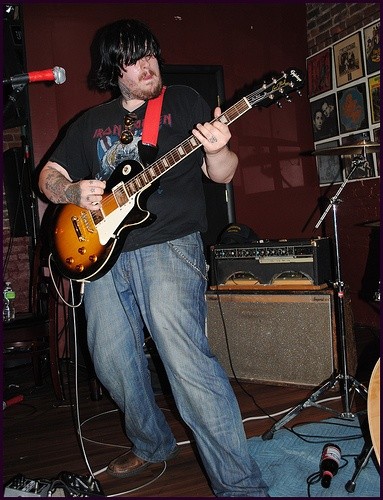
[39,65,310,283]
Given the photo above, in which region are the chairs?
[0,236,67,404]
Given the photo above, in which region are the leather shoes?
[107,450,151,477]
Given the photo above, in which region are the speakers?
[205,285,356,389]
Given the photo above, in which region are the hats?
[216,223,258,245]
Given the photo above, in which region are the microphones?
[352,156,371,171]
[0,66,67,90]
[2,395,24,411]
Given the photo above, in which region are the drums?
[365,354,381,475]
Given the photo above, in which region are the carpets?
[247,411,380,496]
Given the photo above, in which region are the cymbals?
[351,218,383,229]
[311,140,380,155]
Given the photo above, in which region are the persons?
[313,101,338,142]
[37,18,271,497]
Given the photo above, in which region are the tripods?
[261,167,368,441]
